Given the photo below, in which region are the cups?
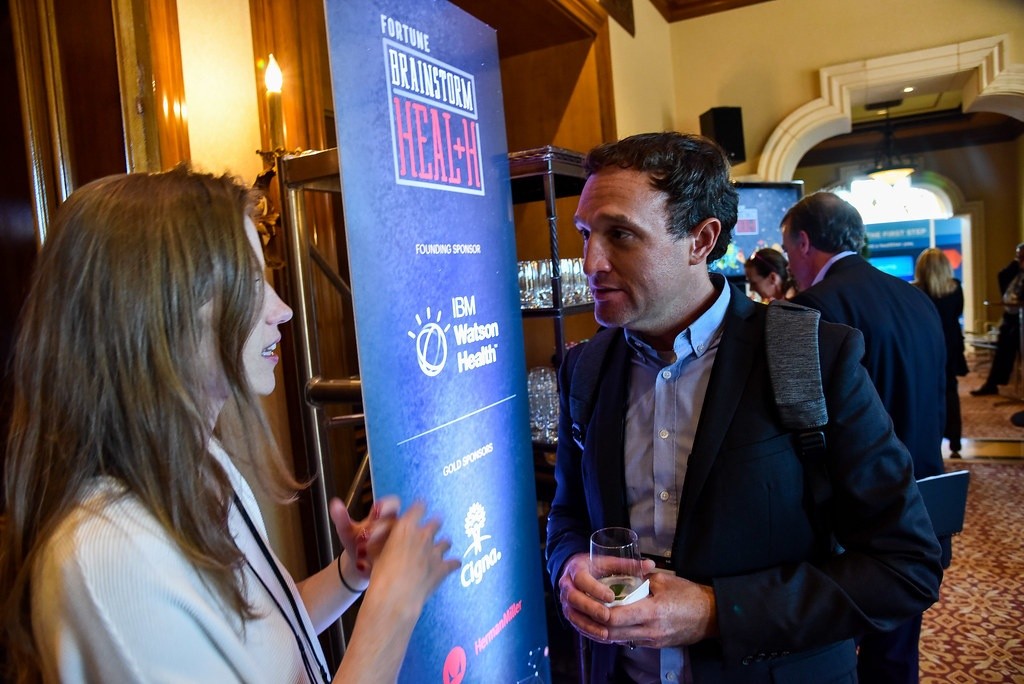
[527,366,560,441]
[590,527,645,606]
[518,258,593,307]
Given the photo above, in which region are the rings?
[629,641,636,651]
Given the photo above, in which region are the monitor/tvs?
[706,179,806,283]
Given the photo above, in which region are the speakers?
[700,107,747,165]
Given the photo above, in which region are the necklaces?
[226,479,331,684]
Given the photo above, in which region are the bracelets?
[338,548,366,593]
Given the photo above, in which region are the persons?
[969,240,1024,396]
[0,160,462,684]
[745,191,970,569]
[546,130,944,684]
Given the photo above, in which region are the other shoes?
[970,384,998,395]
[950,439,961,452]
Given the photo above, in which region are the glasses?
[750,252,769,265]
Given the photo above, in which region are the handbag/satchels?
[1002,275,1024,313]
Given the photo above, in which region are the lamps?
[865,107,916,182]
